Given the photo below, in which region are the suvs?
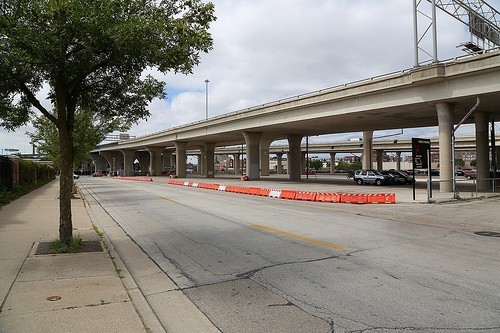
[464,170,477,180]
[354,169,385,186]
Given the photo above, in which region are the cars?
[73,173,79,179]
[379,170,416,185]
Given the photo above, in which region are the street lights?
[29,142,37,156]
[205,80,210,118]
[238,145,243,176]
